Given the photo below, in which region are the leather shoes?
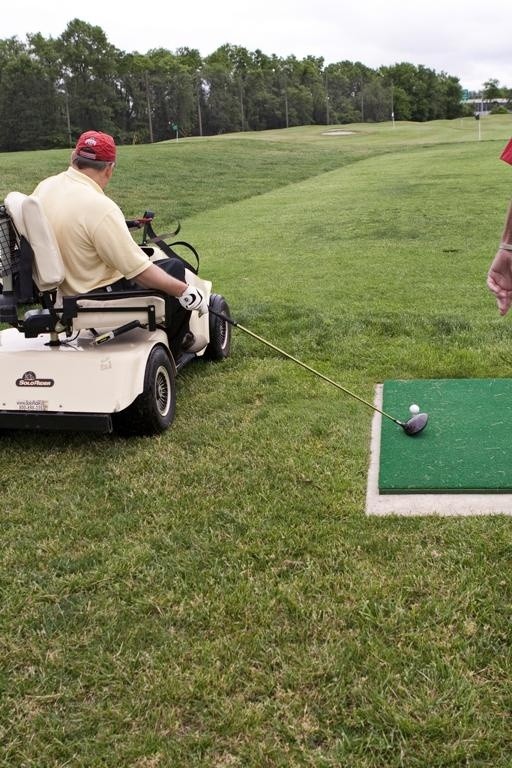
[181,332,208,352]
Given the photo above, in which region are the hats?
[74,129,116,161]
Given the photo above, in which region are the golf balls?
[409,405,419,414]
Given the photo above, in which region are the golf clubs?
[181,295,428,436]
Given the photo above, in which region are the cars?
[0,245,232,437]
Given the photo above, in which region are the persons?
[29,128,210,356]
[487,138,511,315]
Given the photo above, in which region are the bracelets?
[498,242,510,252]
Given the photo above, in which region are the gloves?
[178,283,208,314]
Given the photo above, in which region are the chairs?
[5,191,166,346]
